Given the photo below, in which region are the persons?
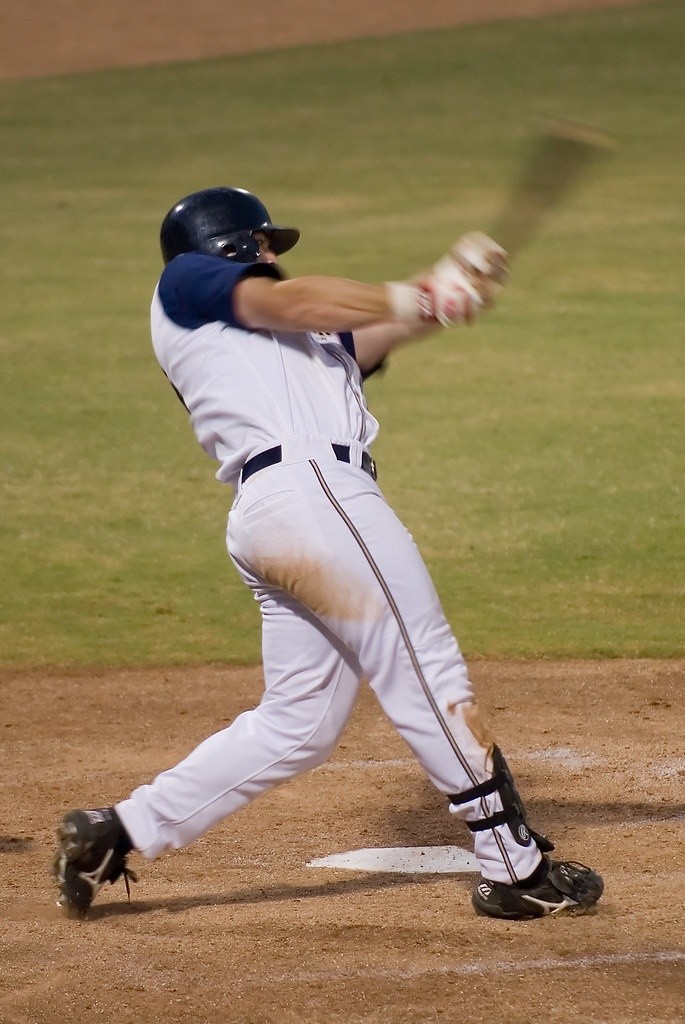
[50,182,609,921]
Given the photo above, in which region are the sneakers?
[472,854,605,921]
[52,805,138,920]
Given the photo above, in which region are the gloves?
[385,234,507,344]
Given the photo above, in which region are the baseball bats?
[483,129,590,247]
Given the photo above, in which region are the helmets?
[160,187,301,263]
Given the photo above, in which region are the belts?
[239,444,378,485]
